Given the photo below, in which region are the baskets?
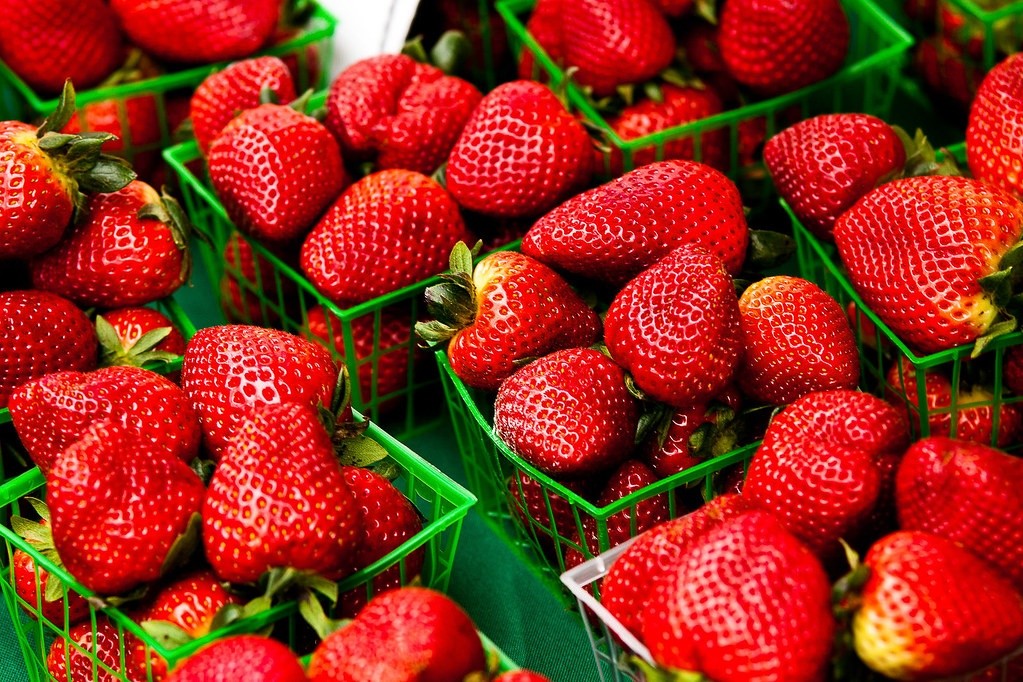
[0,0,1023,682]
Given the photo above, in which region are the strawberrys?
[0,0,1023,682]
